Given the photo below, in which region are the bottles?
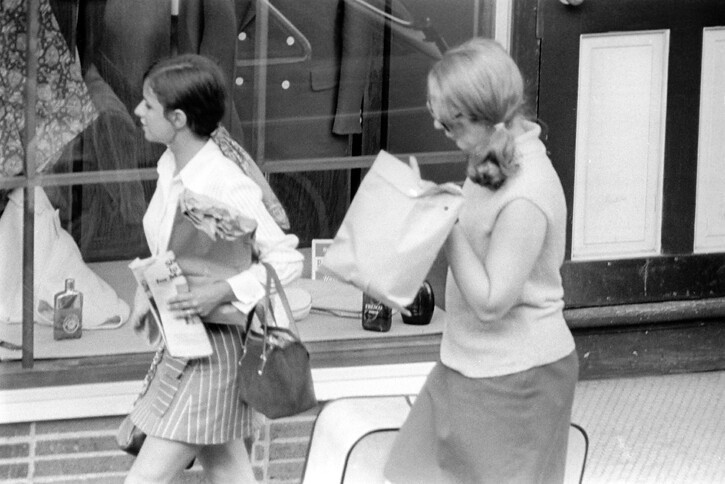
[402,279,435,326]
[362,291,392,332]
[53,278,83,341]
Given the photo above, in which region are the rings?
[187,310,191,317]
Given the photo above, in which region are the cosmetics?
[53,279,84,340]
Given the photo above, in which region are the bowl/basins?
[282,286,312,323]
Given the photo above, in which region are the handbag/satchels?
[321,150,464,316]
[236,258,318,421]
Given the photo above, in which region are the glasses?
[427,100,463,132]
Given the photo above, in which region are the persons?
[125,55,304,484]
[178,0,375,248]
[383,38,578,484]
